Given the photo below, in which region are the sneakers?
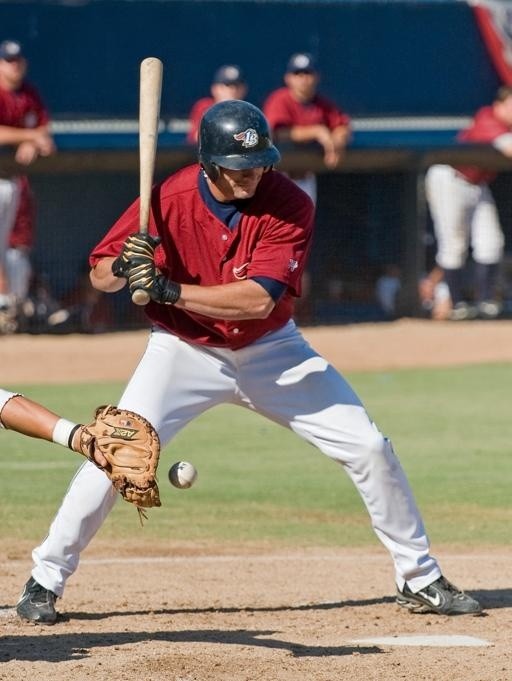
[16,577,57,625]
[398,577,481,614]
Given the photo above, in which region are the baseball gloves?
[72,406,162,507]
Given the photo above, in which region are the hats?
[286,53,317,72]
[0,43,24,63]
[212,65,243,84]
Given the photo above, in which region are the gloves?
[112,232,161,278]
[127,255,181,303]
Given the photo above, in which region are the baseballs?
[169,461,198,488]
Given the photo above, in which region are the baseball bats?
[131,57,163,305]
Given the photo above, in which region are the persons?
[27,262,154,336]
[16,95,484,626]
[297,257,407,327]
[420,92,512,320]
[261,53,351,208]
[1,388,162,507]
[1,37,54,334]
[188,64,250,144]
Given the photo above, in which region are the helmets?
[199,99,282,185]
